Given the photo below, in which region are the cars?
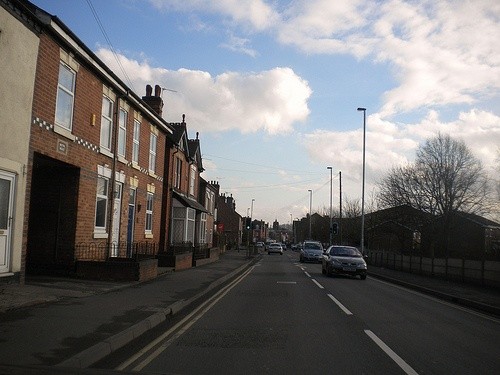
[321,245,369,280]
[267,243,284,255]
[300,241,326,263]
[255,241,303,250]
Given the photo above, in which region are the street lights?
[325,166,333,245]
[250,198,256,244]
[357,106,366,255]
[308,189,312,240]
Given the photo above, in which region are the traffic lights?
[333,222,338,235]
